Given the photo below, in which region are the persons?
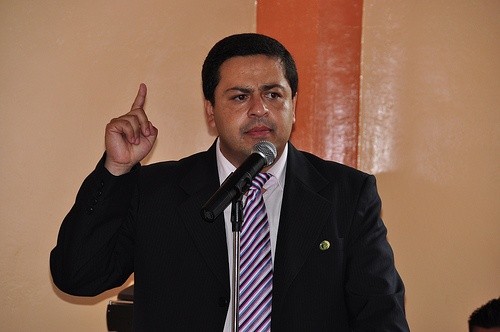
[50,33,410,332]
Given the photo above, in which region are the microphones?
[200,141,277,223]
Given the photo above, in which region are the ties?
[232,174,274,332]
